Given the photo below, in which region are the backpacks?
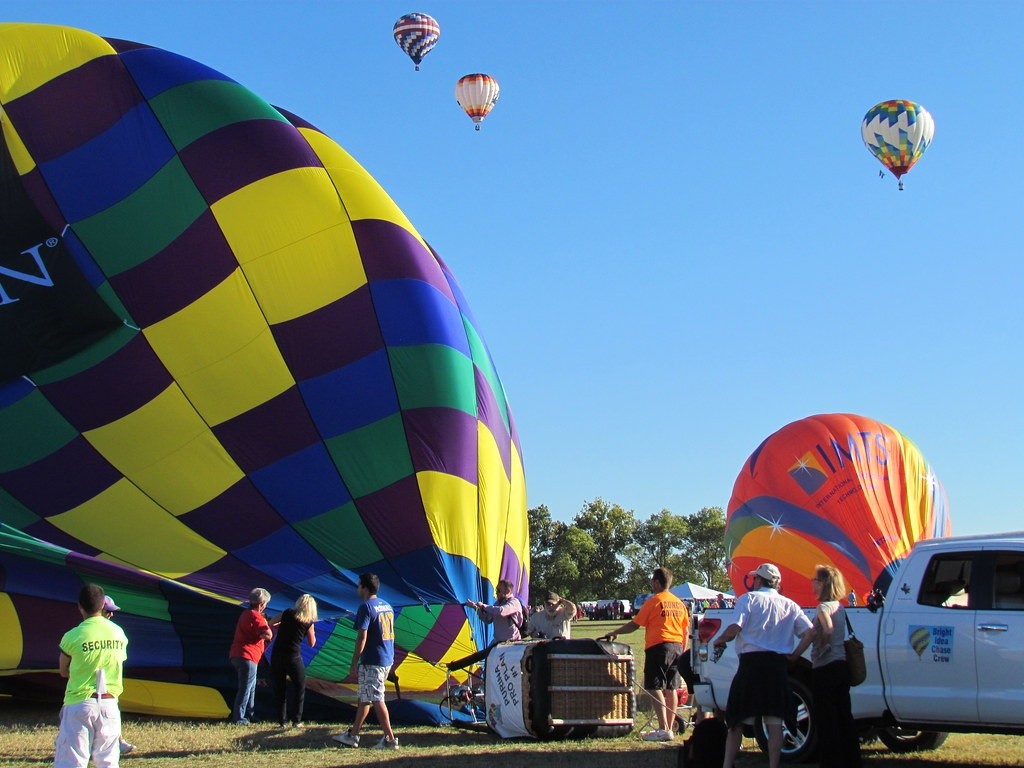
[509,597,529,639]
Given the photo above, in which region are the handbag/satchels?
[844,636,866,686]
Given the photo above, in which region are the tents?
[669,582,736,601]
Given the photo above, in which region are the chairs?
[636,673,694,732]
[993,569,1023,610]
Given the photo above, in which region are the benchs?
[921,571,967,609]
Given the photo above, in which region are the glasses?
[497,588,501,593]
[106,610,115,615]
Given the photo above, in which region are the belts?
[90,692,114,699]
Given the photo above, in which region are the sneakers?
[372,735,399,749]
[332,729,360,748]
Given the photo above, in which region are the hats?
[751,563,781,588]
[716,594,723,597]
[104,595,121,611]
[545,592,560,605]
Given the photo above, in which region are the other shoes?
[644,728,674,741]
[120,739,137,754]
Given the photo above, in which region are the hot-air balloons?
[861,99,935,191]
[724,412,951,609]
[456,74,500,130]
[0,20,638,741]
[393,12,442,72]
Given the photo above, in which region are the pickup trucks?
[691,531,1024,764]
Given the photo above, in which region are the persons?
[716,593,726,609]
[466,579,523,648]
[713,563,817,768]
[605,568,690,741]
[527,593,577,640]
[572,599,625,623]
[269,594,317,728]
[228,588,273,726]
[867,589,886,607]
[848,589,857,607]
[54,584,137,768]
[331,572,399,751]
[684,598,736,616]
[810,564,862,768]
